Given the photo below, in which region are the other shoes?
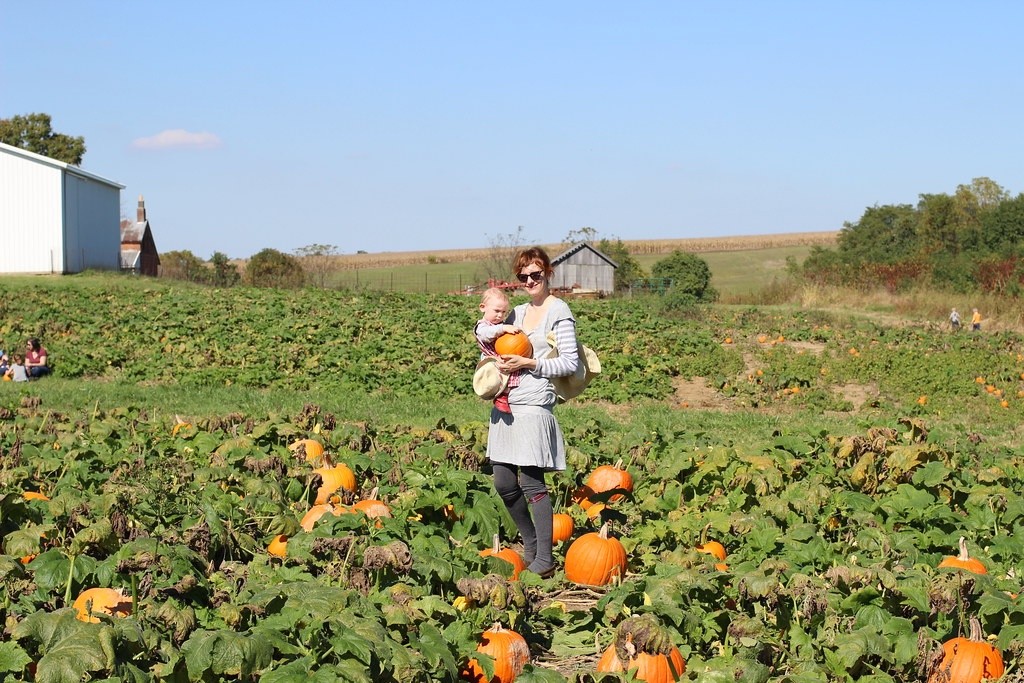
[493,393,511,413]
[526,564,556,578]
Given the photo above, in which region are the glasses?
[516,269,544,282]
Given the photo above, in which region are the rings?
[505,369,507,372]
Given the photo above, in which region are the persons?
[949,307,963,332]
[0,337,53,385]
[972,308,982,331]
[472,246,579,579]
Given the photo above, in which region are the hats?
[547,331,602,402]
[473,356,511,400]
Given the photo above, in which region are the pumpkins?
[13,439,730,683]
[926,617,1004,683]
[725,324,1024,408]
[494,330,532,360]
[938,536,988,574]
